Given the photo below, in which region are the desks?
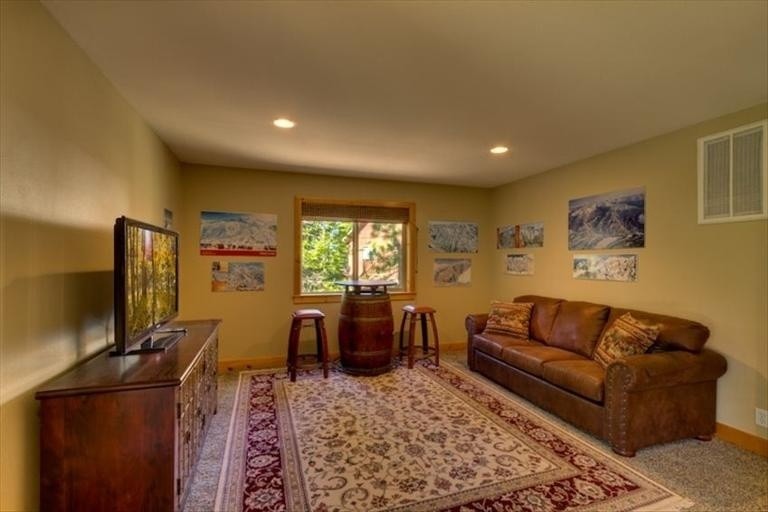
[335,281,396,377]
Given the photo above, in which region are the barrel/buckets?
[338,290,394,377]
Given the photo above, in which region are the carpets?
[213,351,694,512]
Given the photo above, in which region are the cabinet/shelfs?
[34,316,222,511]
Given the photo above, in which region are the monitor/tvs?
[110,216,187,356]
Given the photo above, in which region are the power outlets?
[755,407,768,428]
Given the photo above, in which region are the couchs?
[464,293,728,456]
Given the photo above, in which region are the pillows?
[592,311,661,368]
[482,296,535,345]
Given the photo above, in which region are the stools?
[398,304,439,368]
[286,308,329,382]
